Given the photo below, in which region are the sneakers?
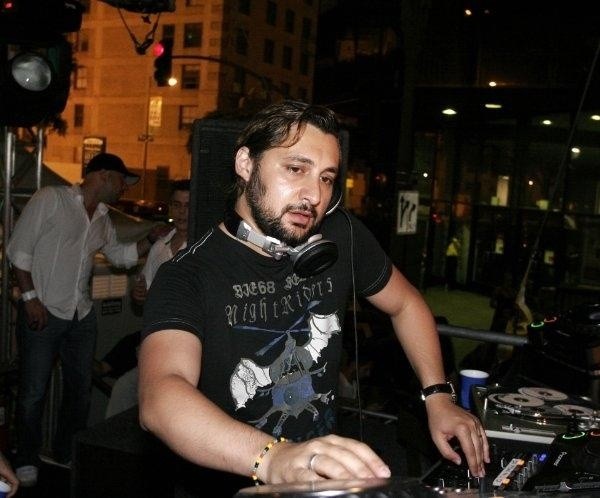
[13,449,73,487]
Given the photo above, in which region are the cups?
[458,370,489,410]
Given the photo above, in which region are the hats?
[85,151,141,187]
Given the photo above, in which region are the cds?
[496,386,597,416]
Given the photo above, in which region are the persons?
[135,95,493,495]
[0,454,22,497]
[95,177,189,420]
[0,151,173,488]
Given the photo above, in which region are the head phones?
[223,180,339,279]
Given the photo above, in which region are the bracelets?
[420,380,457,406]
[20,291,39,303]
[249,437,289,486]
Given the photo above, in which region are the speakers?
[188,116,347,245]
[73,406,175,498]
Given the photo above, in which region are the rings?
[310,453,319,468]
